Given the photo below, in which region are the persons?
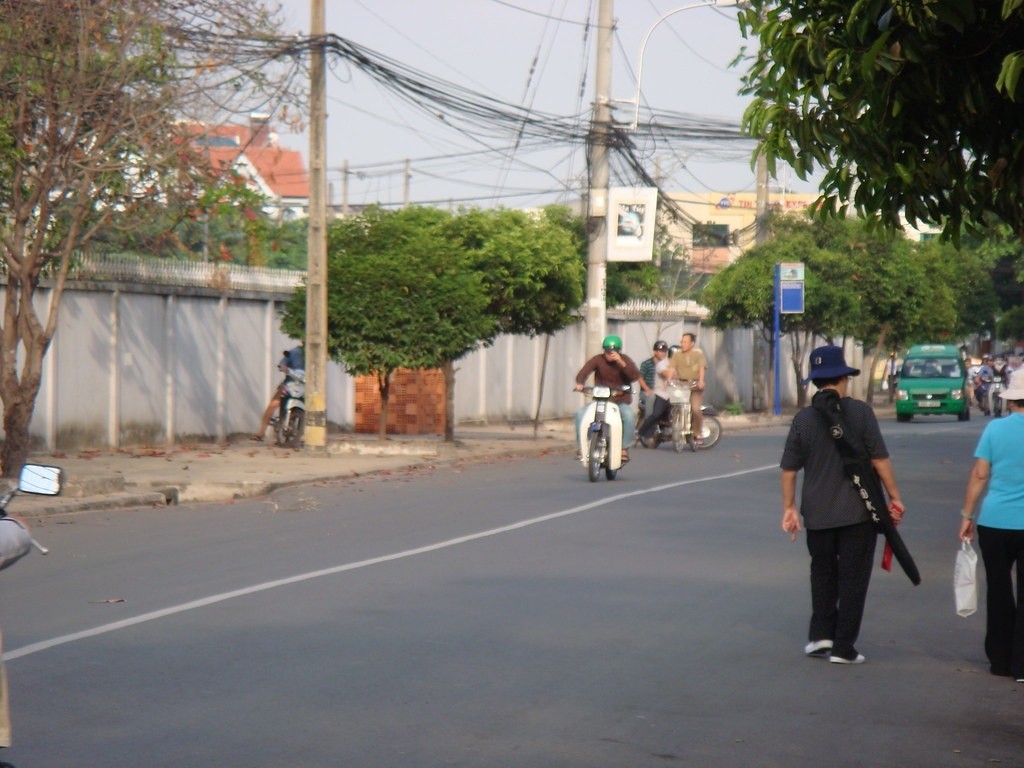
[575,336,640,463]
[884,355,898,403]
[0,513,32,573]
[780,345,905,665]
[251,338,305,441]
[965,355,1013,415]
[959,363,1023,682]
[635,333,706,447]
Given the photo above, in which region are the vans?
[894,343,973,423]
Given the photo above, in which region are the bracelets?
[960,510,974,520]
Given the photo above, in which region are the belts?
[679,378,699,382]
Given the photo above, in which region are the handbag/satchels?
[882,380,889,391]
[954,536,978,617]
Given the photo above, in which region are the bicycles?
[661,378,698,453]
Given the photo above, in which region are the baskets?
[668,385,690,405]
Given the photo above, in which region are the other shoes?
[247,434,265,443]
[694,434,704,445]
[638,432,648,448]
[805,639,833,655]
[830,654,866,664]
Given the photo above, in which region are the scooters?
[633,388,724,452]
[268,349,306,449]
[970,368,1014,417]
[572,385,637,482]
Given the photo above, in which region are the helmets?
[654,340,668,351]
[603,336,623,350]
[668,345,681,357]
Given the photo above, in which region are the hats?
[802,346,860,384]
[998,369,1024,400]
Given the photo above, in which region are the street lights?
[580,0,746,414]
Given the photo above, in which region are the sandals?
[621,448,630,463]
